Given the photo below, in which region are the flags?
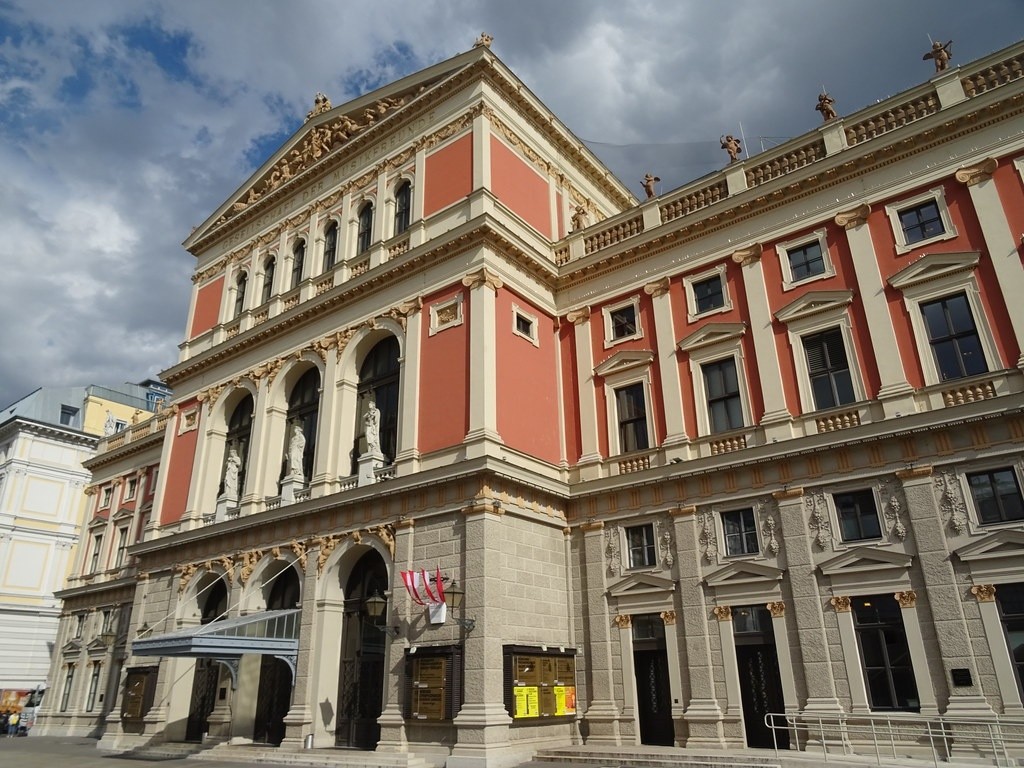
[401,567,445,604]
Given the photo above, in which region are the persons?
[240,540,308,581]
[177,554,234,589]
[285,425,306,475]
[815,92,836,121]
[720,134,741,162]
[316,525,395,570]
[924,40,950,72]
[641,173,658,199]
[363,401,381,452]
[570,206,587,232]
[215,91,407,228]
[224,450,240,494]
[8,711,19,738]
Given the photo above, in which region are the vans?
[18,687,46,734]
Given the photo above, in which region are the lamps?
[136,622,154,639]
[100,627,130,660]
[441,578,476,633]
[365,588,399,639]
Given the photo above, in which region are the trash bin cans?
[306,735,313,749]
[202,733,208,744]
